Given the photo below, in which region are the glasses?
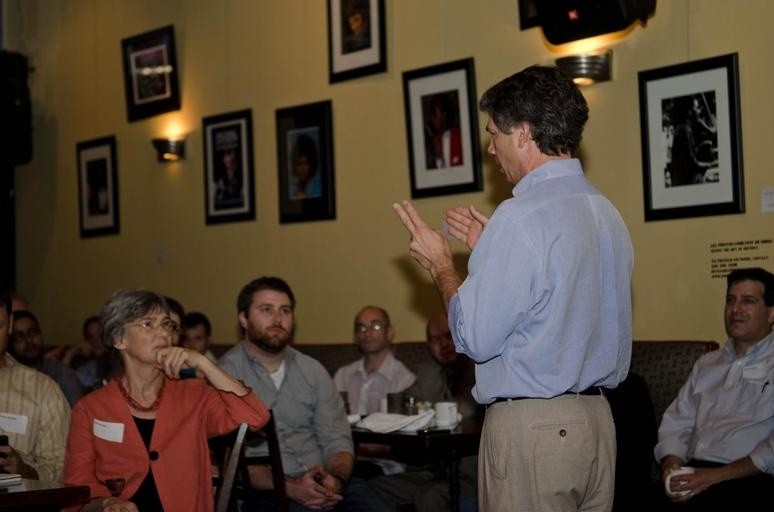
[127,319,177,333]
[357,323,389,333]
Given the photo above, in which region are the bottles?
[404,396,416,414]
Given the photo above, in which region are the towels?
[356,407,436,434]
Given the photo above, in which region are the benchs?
[310,342,724,509]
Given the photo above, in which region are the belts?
[488,387,600,405]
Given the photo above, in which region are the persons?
[217,277,356,512]
[389,62,637,512]
[662,89,720,187]
[651,265,774,512]
[344,6,368,50]
[2,289,271,512]
[336,307,482,511]
[211,130,245,212]
[429,98,464,169]
[287,130,322,202]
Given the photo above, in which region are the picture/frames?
[275,97,335,223]
[203,105,256,224]
[399,55,483,202]
[635,50,747,225]
[326,0,389,86]
[76,134,119,238]
[119,24,182,121]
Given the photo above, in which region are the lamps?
[546,36,622,82]
[150,118,193,169]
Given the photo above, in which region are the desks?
[0,474,92,512]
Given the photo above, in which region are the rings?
[689,490,695,496]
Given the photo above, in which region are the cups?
[434,400,458,430]
[664,465,695,496]
[386,393,405,414]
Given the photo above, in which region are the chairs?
[231,409,295,512]
[206,422,248,512]
